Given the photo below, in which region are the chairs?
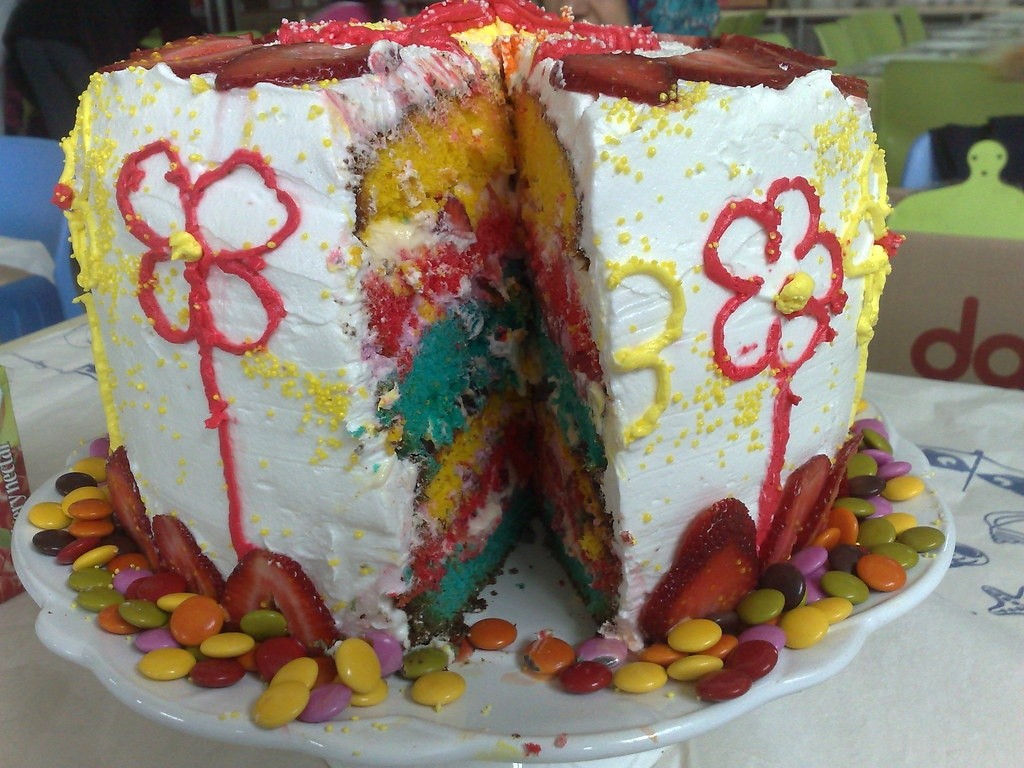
[750,7,932,69]
[0,135,80,319]
[710,9,766,39]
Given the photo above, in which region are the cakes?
[24,0,946,728]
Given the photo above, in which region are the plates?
[9,397,954,768]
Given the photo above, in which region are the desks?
[0,235,65,341]
[874,13,1024,187]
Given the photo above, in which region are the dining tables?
[0,311,1023,768]
